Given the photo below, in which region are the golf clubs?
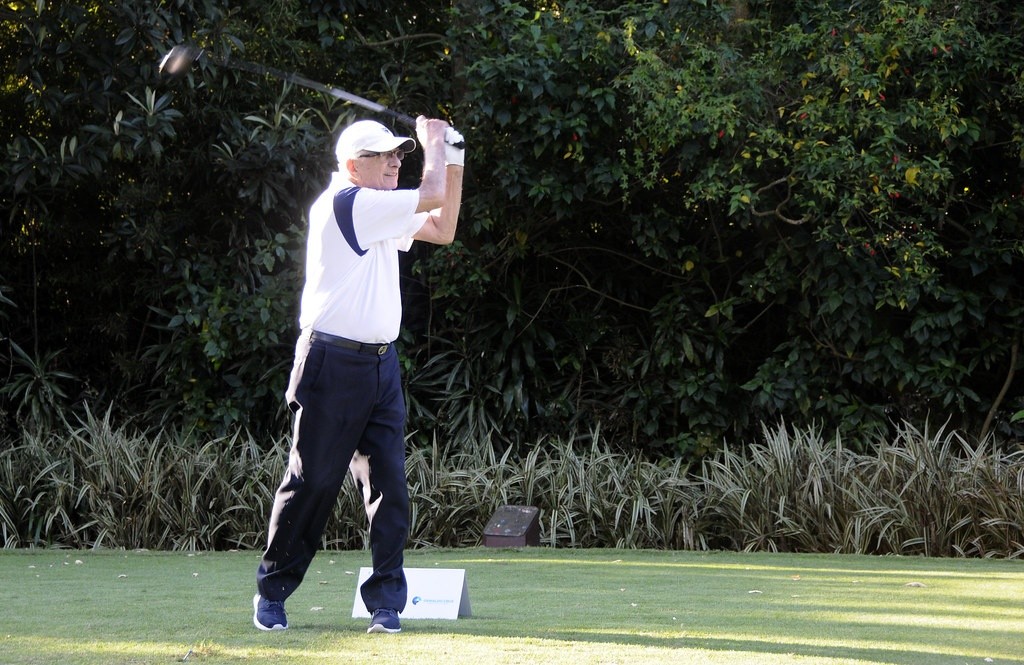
[157,43,474,154]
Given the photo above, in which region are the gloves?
[443,125,466,167]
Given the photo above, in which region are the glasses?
[357,149,405,160]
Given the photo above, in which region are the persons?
[254,115,464,634]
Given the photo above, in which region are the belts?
[301,328,392,356]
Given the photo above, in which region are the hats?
[335,120,417,163]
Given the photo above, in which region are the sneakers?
[366,608,402,633]
[253,591,289,631]
